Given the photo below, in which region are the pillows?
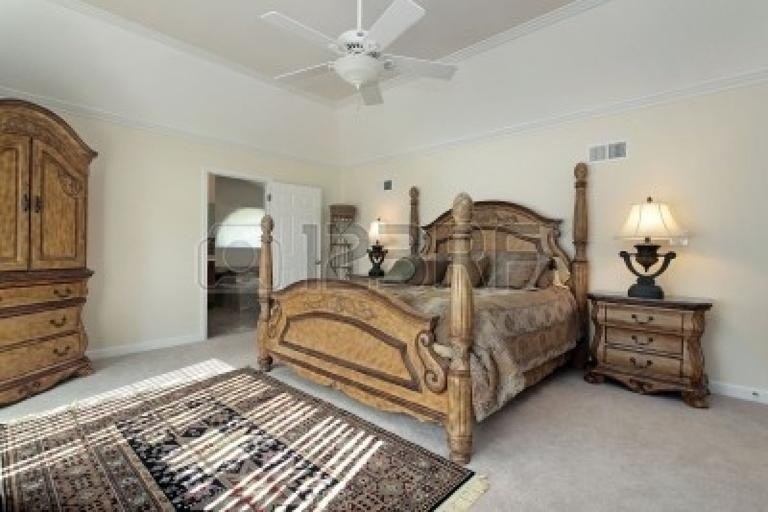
[383,253,551,290]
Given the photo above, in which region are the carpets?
[0,356,490,511]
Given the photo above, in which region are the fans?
[259,0,456,90]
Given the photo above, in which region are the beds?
[258,161,589,466]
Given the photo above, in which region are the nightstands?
[350,273,385,288]
[586,292,713,408]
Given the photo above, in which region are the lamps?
[329,205,356,280]
[333,55,384,90]
[367,222,388,277]
[619,196,684,298]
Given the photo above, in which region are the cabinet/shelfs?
[0,97,98,272]
[0,270,94,406]
